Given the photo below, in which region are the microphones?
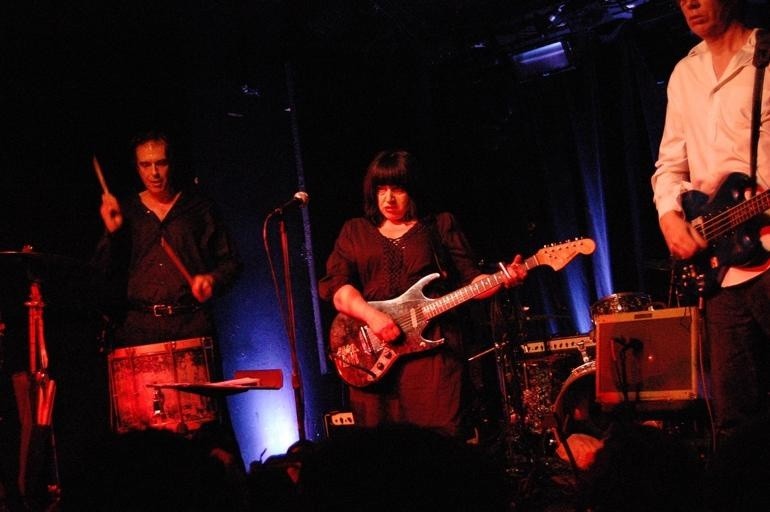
[267,190,310,223]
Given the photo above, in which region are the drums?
[515,350,580,435]
[590,292,653,317]
[550,361,662,471]
[108,337,216,433]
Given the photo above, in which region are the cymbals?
[473,315,558,327]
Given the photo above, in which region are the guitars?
[330,236,596,387]
[668,172,770,300]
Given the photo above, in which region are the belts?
[131,303,201,318]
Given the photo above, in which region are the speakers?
[595,307,699,402]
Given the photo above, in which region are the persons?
[318,147,527,433]
[651,1,770,432]
[81,132,241,344]
